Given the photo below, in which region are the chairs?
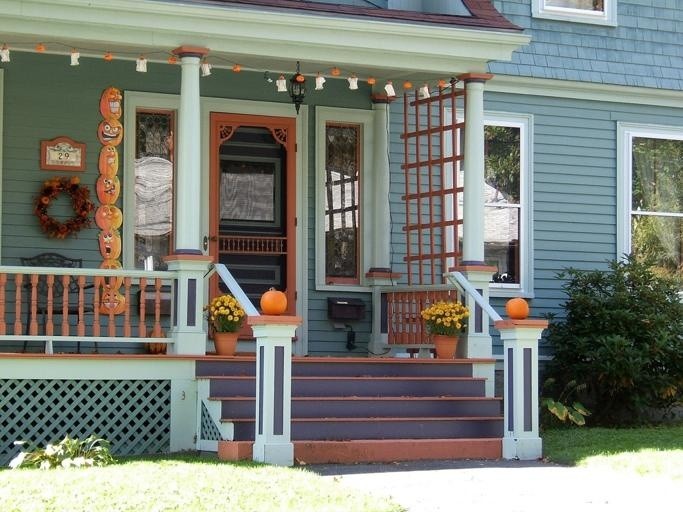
[16,251,101,356]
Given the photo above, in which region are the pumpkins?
[95,87,126,316]
[260,286,288,315]
[506,296,530,319]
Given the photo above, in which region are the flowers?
[201,292,246,334]
[419,298,472,337]
[30,174,95,243]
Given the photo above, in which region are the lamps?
[287,57,306,116]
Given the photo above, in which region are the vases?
[432,332,459,361]
[212,331,239,357]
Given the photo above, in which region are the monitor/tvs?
[219,154,282,229]
